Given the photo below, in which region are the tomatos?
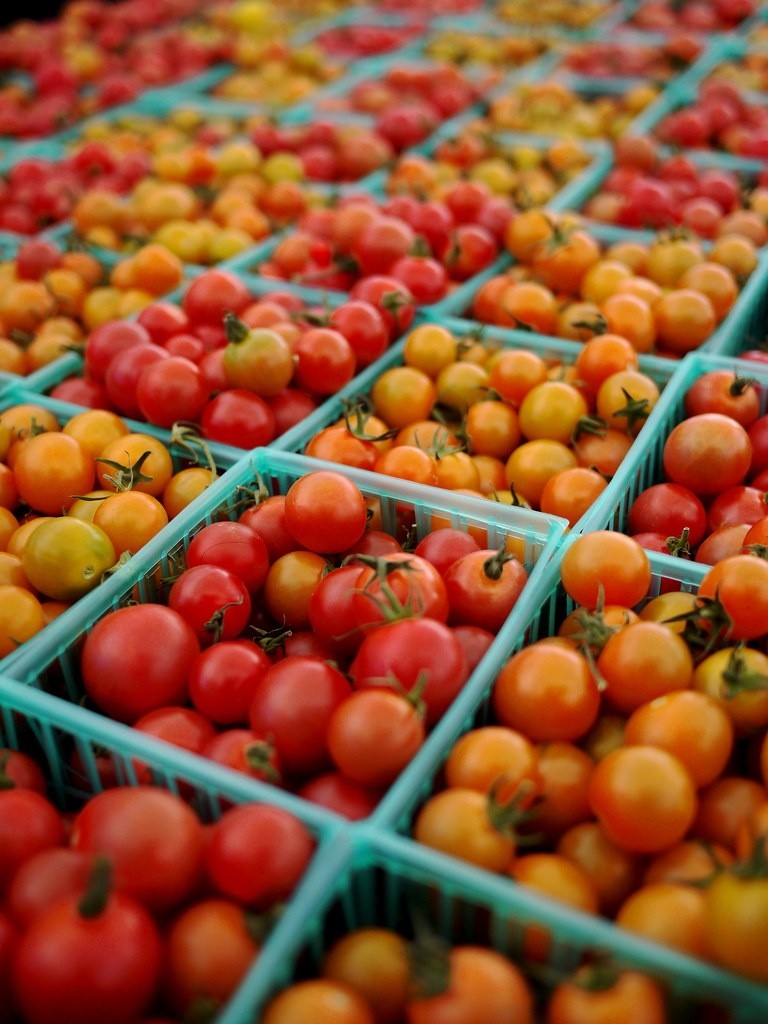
[0,0,768,1024]
